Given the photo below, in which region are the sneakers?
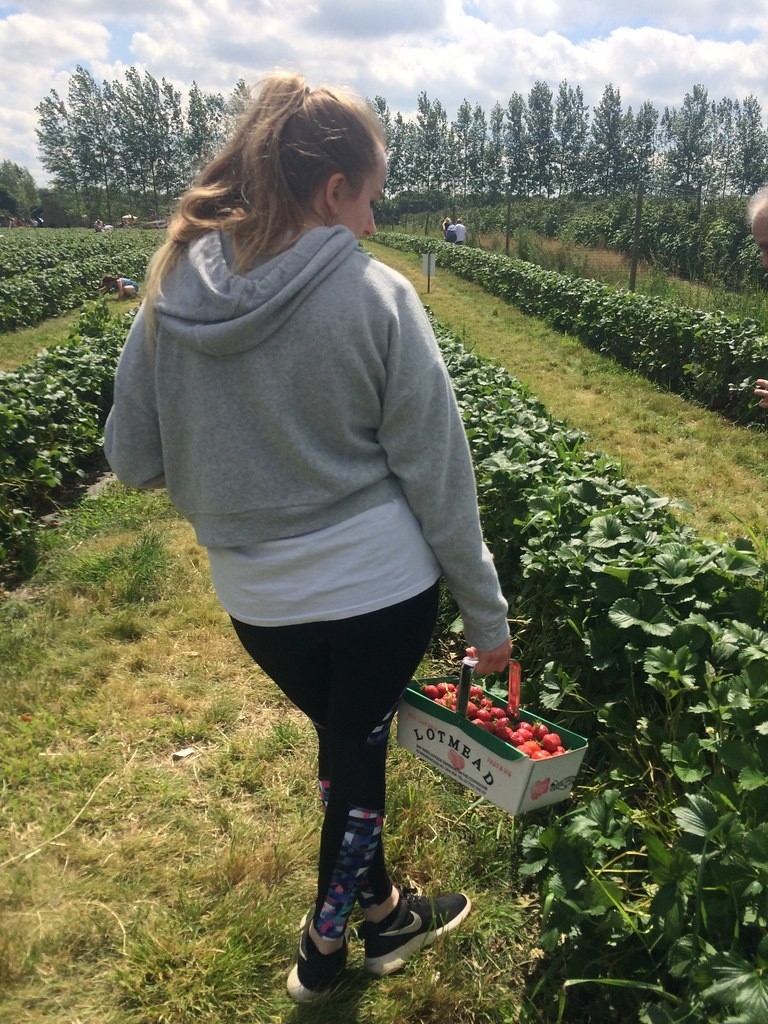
[359,889,470,979]
[286,905,351,1004]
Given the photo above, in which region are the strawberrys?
[419,682,568,761]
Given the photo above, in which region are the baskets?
[396,659,589,817]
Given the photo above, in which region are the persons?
[94,218,113,232]
[442,217,466,246]
[9,217,44,229]
[104,79,515,1005]
[99,275,138,302]
